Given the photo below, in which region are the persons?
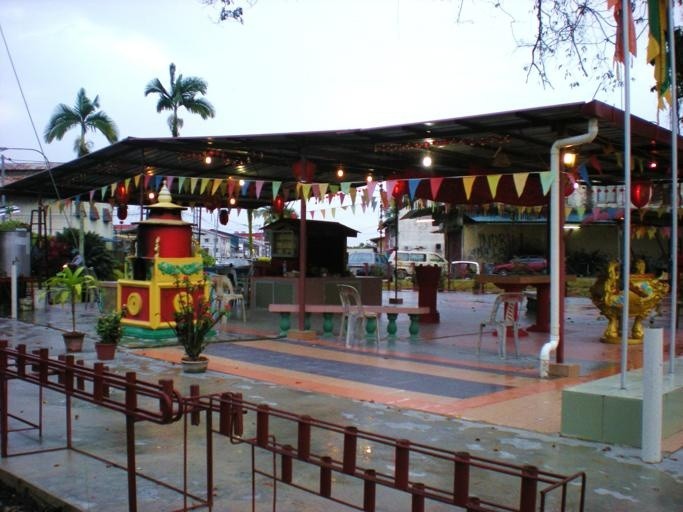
[433,244,442,256]
[60,249,82,271]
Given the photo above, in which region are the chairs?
[228,264,252,308]
[211,274,248,325]
[331,283,385,349]
[477,292,529,360]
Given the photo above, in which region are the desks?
[267,299,430,340]
[473,270,578,338]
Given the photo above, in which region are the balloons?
[629,174,653,211]
[270,193,283,217]
[116,204,127,224]
[219,208,229,226]
[389,178,406,199]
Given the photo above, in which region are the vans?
[387,249,451,279]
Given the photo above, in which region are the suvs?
[348,252,393,282]
[492,254,548,277]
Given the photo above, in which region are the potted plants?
[92,313,123,359]
[38,263,106,352]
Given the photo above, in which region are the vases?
[178,355,209,374]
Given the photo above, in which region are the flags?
[645,0,670,110]
[607,0,639,77]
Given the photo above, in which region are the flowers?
[163,272,229,361]
